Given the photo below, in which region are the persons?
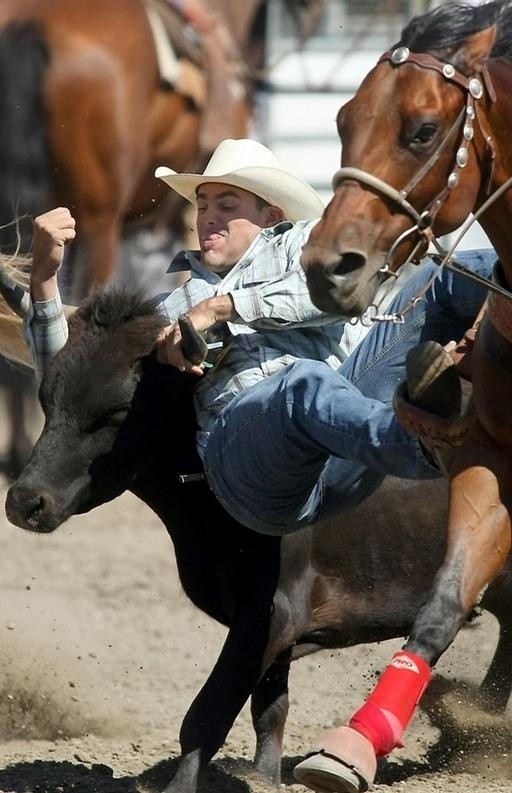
[23,137,505,536]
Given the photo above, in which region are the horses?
[292,0,512,793]
[0,0,270,485]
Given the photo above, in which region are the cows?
[0,278,512,793]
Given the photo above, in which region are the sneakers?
[405,341,462,476]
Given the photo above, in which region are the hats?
[154,139,325,222]
